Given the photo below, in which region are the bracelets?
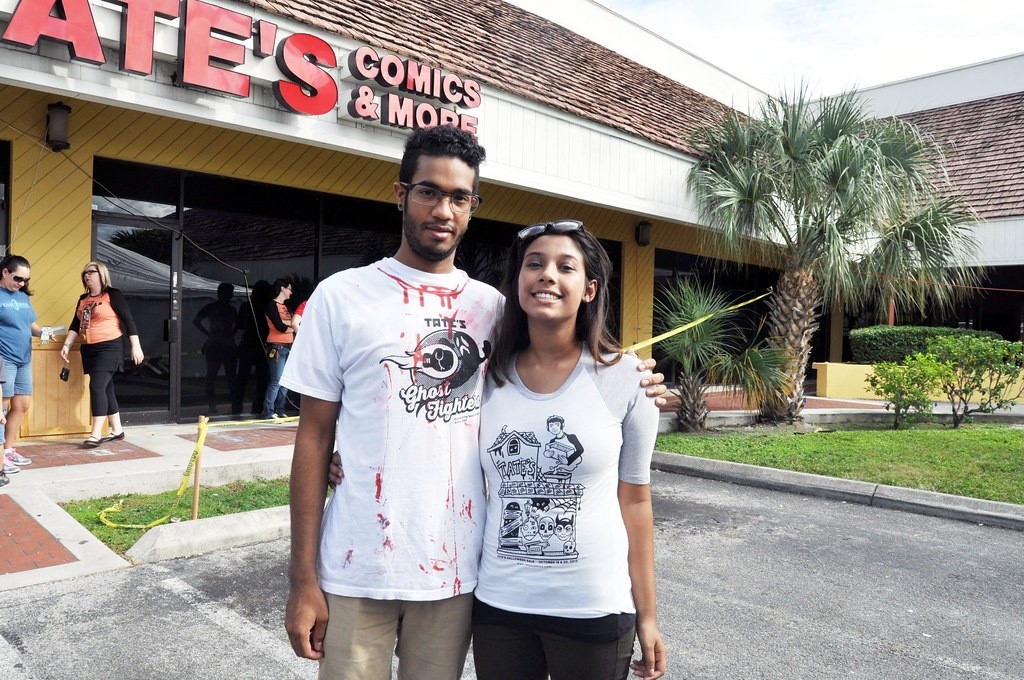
[63,344,70,349]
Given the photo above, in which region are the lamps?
[634,221,652,247]
[45,100,73,153]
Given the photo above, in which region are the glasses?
[83,270,99,275]
[399,181,480,214]
[517,219,586,252]
[284,287,292,292]
[8,270,30,283]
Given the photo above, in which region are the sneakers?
[2,461,20,474]
[5,448,31,466]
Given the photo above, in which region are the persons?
[278,123,667,680]
[263,278,308,419]
[60,261,144,446]
[192,279,273,416]
[0,254,57,487]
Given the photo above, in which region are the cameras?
[60,367,70,382]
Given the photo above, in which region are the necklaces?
[84,286,105,309]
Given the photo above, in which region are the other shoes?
[84,436,103,446]
[103,431,124,442]
[270,413,279,419]
[0,471,10,487]
[280,414,288,418]
